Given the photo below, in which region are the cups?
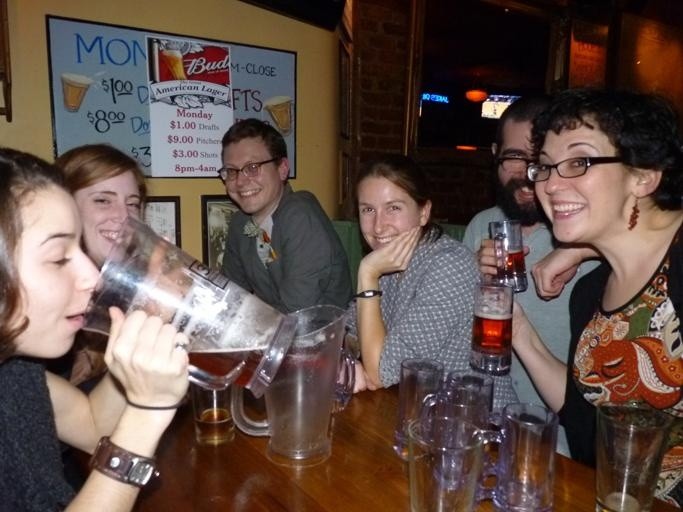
[494,153,535,174]
[218,157,282,181]
[527,155,630,183]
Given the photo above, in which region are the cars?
[392,357,444,460]
[79,216,300,400]
[407,415,503,512]
[438,369,495,447]
[419,387,491,486]
[331,347,355,413]
[468,281,514,377]
[592,400,674,512]
[488,219,528,293]
[228,304,349,469]
[492,401,559,511]
[478,428,501,504]
[189,382,235,447]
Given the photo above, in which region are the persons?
[462,94,602,457]
[52,144,145,270]
[218,119,351,336]
[0,146,188,512]
[335,155,520,423]
[482,88,682,508]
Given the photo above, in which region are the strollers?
[243,220,270,244]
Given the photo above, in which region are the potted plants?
[198,194,239,269]
[138,194,181,254]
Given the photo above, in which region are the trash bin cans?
[350,289,381,298]
[87,435,155,488]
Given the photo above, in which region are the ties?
[481,94,522,120]
